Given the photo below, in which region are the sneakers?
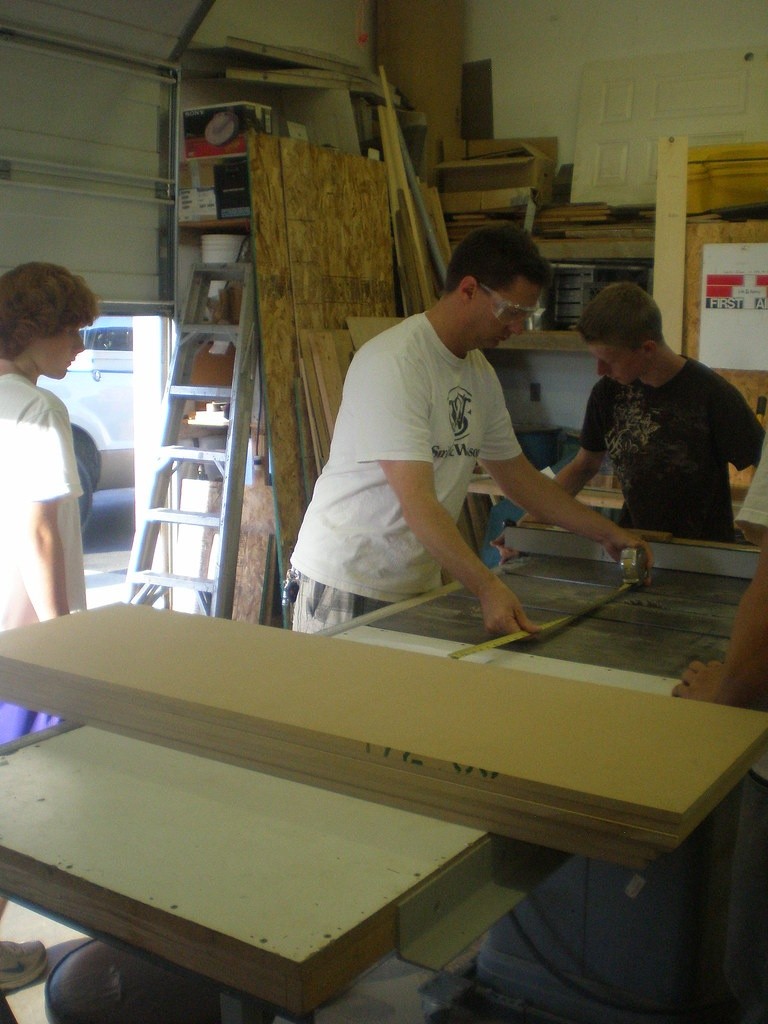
[0,939,48,991]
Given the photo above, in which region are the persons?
[489,282,766,572]
[674,427,768,1024]
[0,261,102,998]
[289,221,656,642]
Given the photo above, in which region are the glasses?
[477,280,545,326]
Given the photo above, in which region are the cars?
[35,313,133,531]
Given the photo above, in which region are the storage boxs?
[431,134,559,214]
[181,133,254,221]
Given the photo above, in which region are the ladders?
[121,260,257,620]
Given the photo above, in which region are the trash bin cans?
[476,808,727,1024]
[43,938,313,1024]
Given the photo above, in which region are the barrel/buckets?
[201,233,245,263]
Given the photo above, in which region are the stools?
[45,942,222,1023]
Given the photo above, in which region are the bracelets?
[503,519,528,557]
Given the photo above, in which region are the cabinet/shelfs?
[166,98,271,616]
[446,233,656,354]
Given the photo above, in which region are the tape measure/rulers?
[446,547,649,660]
